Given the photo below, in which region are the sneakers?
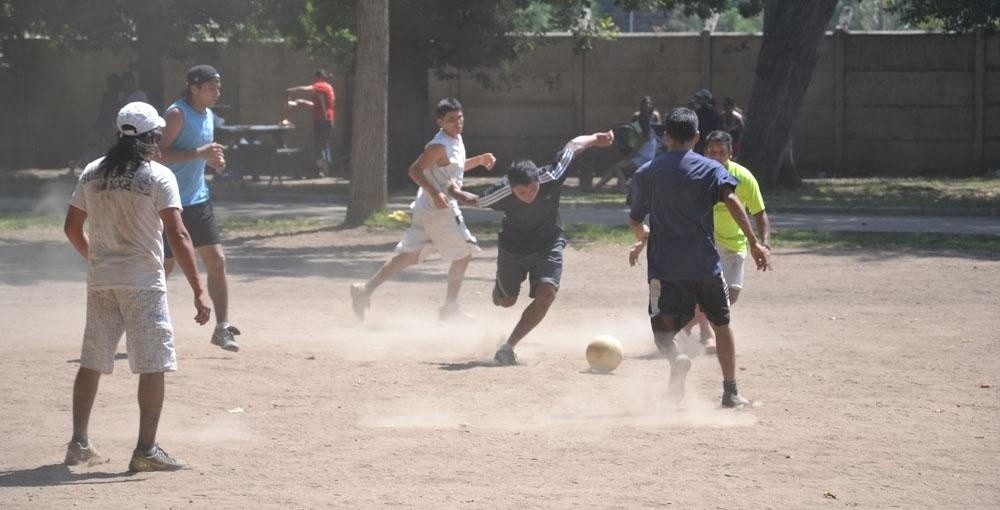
[439,311,467,326]
[669,354,691,397]
[348,281,372,324]
[63,442,99,468]
[132,446,181,478]
[212,327,235,348]
[722,393,752,407]
[700,334,717,353]
[491,349,514,366]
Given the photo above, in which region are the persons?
[285,71,336,179]
[626,113,650,153]
[628,108,775,408]
[351,98,496,337]
[630,130,770,354]
[447,129,615,365]
[113,73,151,119]
[584,115,668,193]
[633,96,662,134]
[719,96,746,160]
[160,64,241,352]
[64,102,213,474]
[693,89,720,156]
[93,74,121,147]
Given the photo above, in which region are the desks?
[214,125,302,179]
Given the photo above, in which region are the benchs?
[269,148,302,185]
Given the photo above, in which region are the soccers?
[586,336,622,372]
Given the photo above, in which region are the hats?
[180,64,220,96]
[116,102,168,133]
[695,90,712,100]
